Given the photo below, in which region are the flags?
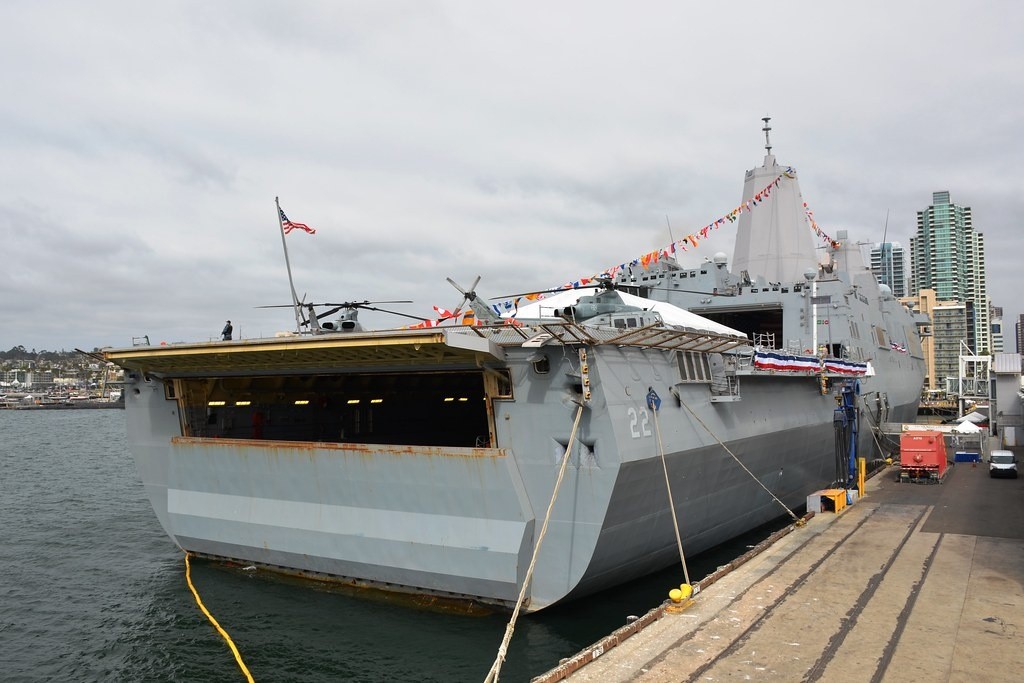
[623,167,795,270]
[409,298,521,330]
[804,201,842,250]
[276,204,316,234]
[526,263,625,301]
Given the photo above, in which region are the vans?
[988,450,1019,478]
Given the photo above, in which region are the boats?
[94,114,933,621]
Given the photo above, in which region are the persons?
[222,321,232,340]
[818,344,828,354]
